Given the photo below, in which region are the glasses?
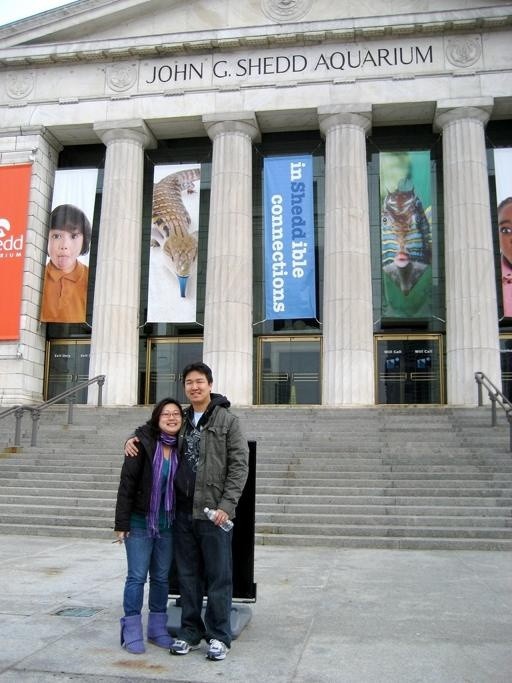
[161,411,182,417]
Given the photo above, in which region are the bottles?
[203,506,234,534]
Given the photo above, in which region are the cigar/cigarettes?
[112,537,126,544]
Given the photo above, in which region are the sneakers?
[204,639,232,662]
[167,636,202,656]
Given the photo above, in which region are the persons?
[109,398,183,653]
[40,203,92,323]
[123,361,249,660]
[497,195,512,316]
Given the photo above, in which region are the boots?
[118,614,145,656]
[145,612,176,651]
